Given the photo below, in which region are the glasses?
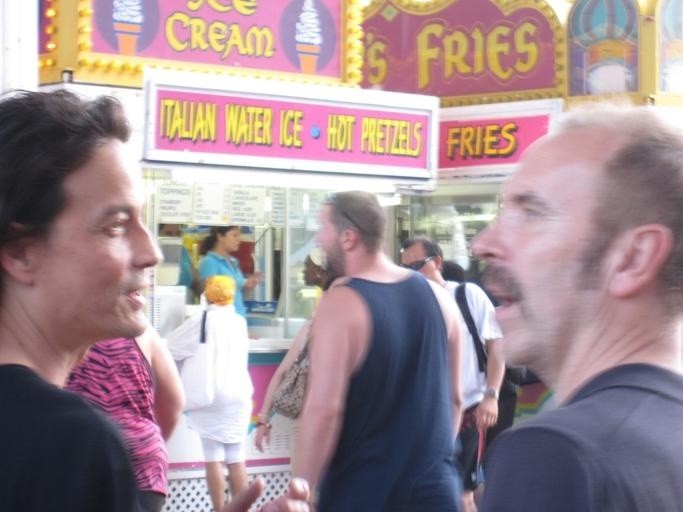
[399,256,434,271]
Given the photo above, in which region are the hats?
[205,275,236,305]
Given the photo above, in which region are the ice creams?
[112,0,143,55]
[295,2,323,75]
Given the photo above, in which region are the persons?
[1,87,310,511]
[157,222,198,294]
[163,275,251,512]
[470,105,679,510]
[60,318,185,512]
[194,225,261,317]
[253,188,525,512]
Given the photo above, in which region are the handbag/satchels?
[273,350,310,420]
[485,380,517,448]
[178,343,216,413]
